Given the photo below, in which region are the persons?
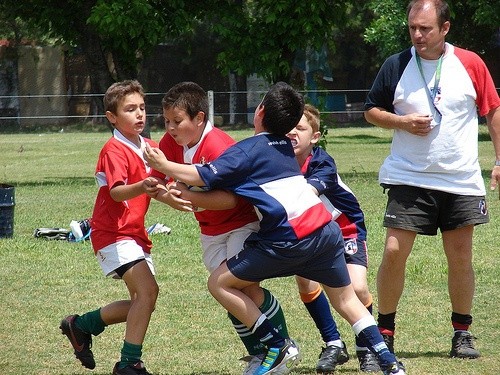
[146,82,300,375]
[362,0,500,359]
[285,102,379,374]
[141,81,407,375]
[59,78,162,375]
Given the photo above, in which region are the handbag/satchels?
[35,227,68,239]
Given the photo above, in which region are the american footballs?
[171,162,209,211]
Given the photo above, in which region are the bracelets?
[494,161,500,166]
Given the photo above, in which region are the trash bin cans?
[0,184,16,238]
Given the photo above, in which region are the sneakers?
[377,323,396,354]
[112,360,152,375]
[378,355,406,375]
[253,339,299,375]
[316,341,349,373]
[59,314,95,370]
[450,330,481,360]
[267,349,302,375]
[356,345,380,373]
[242,353,266,375]
